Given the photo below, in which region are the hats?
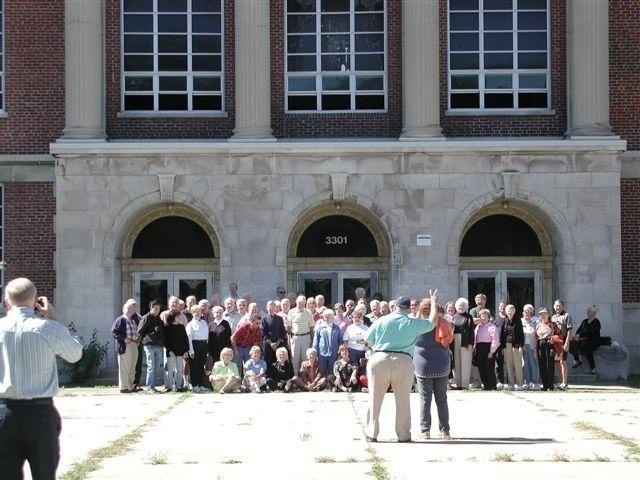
[539,310,547,314]
[396,295,411,310]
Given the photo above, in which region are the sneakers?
[120,384,367,399]
[573,361,581,368]
[438,431,450,440]
[591,368,597,374]
[450,382,569,392]
[418,432,430,439]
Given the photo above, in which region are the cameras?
[35,302,44,313]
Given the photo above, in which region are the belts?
[0,398,54,405]
[194,340,206,343]
[375,349,410,355]
[131,340,138,343]
[293,333,310,336]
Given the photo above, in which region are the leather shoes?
[398,438,411,442]
[366,436,377,442]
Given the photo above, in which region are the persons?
[0,278,84,480]
[363,288,439,444]
[569,303,601,375]
[411,297,453,440]
